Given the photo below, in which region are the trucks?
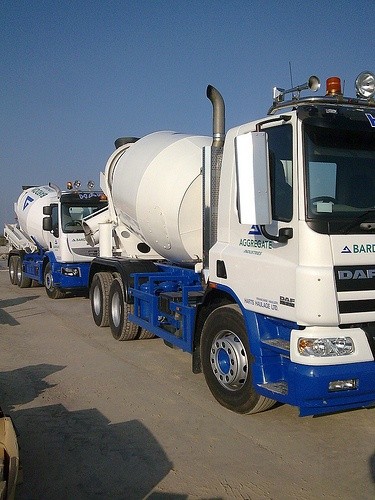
[3,182,108,298]
[78,72,375,416]
